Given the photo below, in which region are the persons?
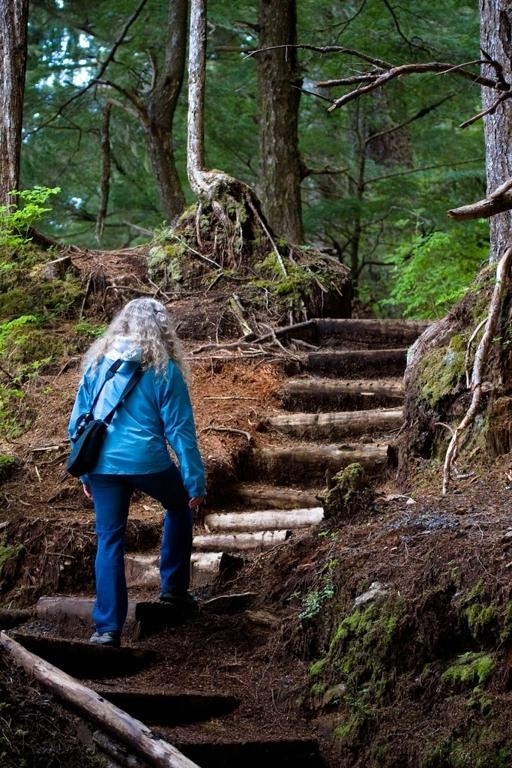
[68,296,208,646]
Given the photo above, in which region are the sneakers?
[90,630,122,646]
[160,589,196,604]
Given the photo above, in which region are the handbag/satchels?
[65,360,143,477]
